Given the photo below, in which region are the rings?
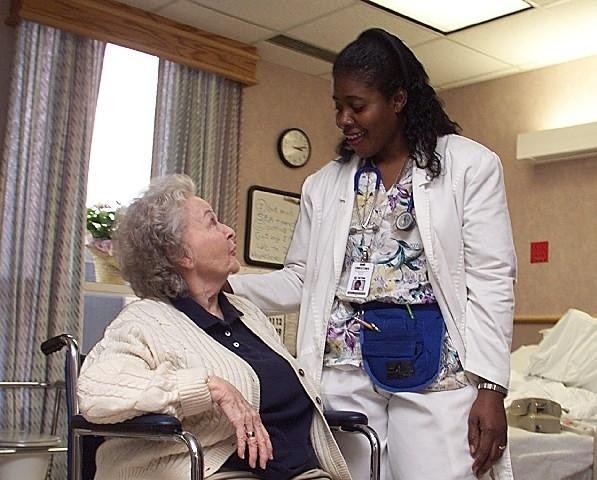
[499,445,506,451]
[246,431,255,438]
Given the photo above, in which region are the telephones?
[505,398,561,433]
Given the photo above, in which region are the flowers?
[86,202,126,248]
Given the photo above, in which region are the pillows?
[526,307,597,393]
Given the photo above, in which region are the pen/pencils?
[371,323,381,332]
[353,316,373,330]
[406,303,415,319]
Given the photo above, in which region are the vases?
[88,249,124,285]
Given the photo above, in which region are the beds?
[505,344,597,480]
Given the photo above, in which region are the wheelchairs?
[39,332,381,480]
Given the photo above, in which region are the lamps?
[516,123,597,167]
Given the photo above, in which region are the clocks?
[278,128,312,168]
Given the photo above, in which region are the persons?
[73,172,352,480]
[222,27,518,480]
[354,280,361,290]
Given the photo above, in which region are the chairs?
[0,382,61,480]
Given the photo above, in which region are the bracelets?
[475,382,508,400]
